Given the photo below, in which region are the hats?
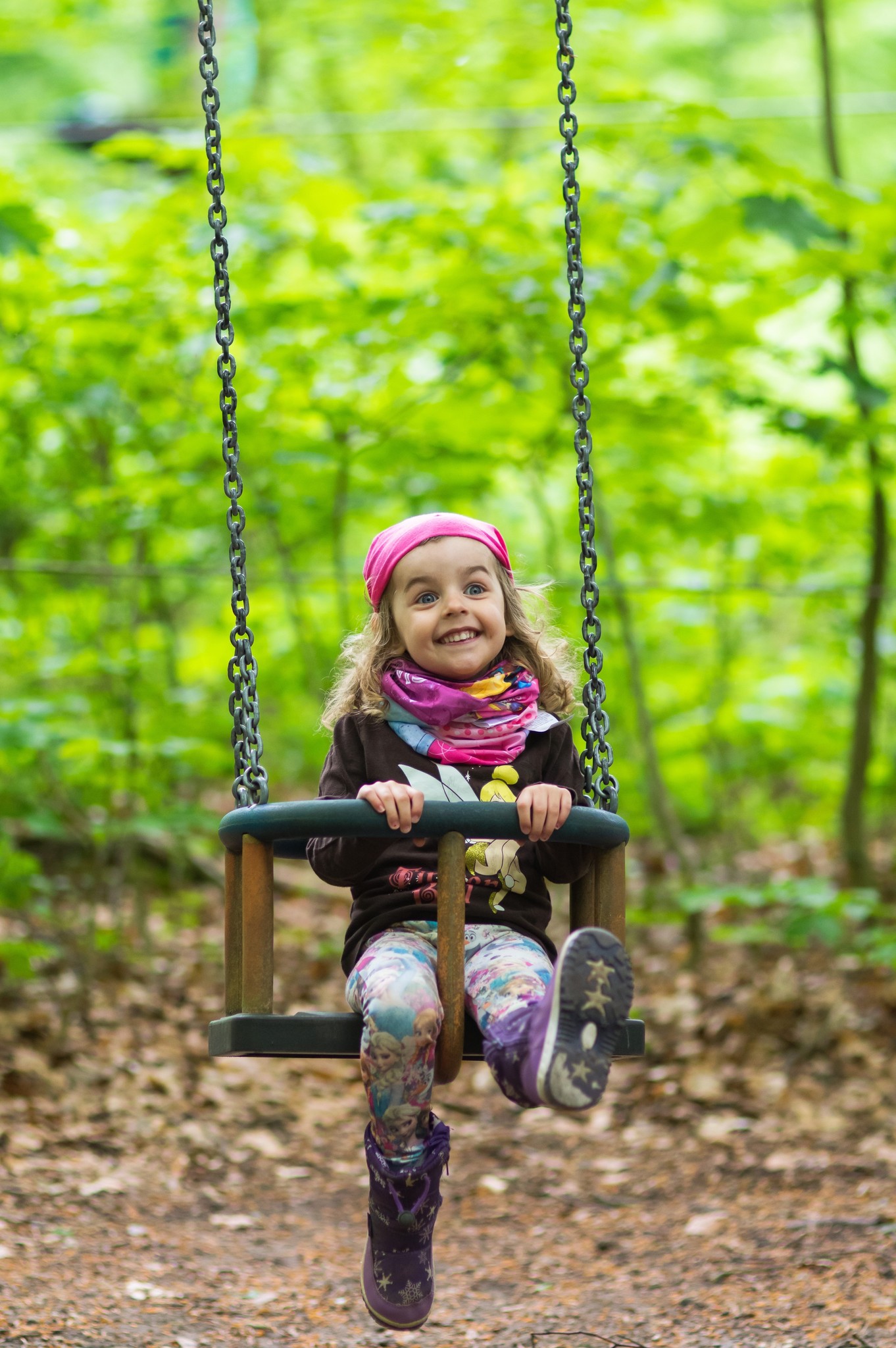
[363,510,515,610]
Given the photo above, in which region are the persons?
[305,513,634,1339]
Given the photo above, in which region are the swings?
[193,0,661,1075]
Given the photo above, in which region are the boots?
[362,1112,452,1331]
[519,923,635,1114]
